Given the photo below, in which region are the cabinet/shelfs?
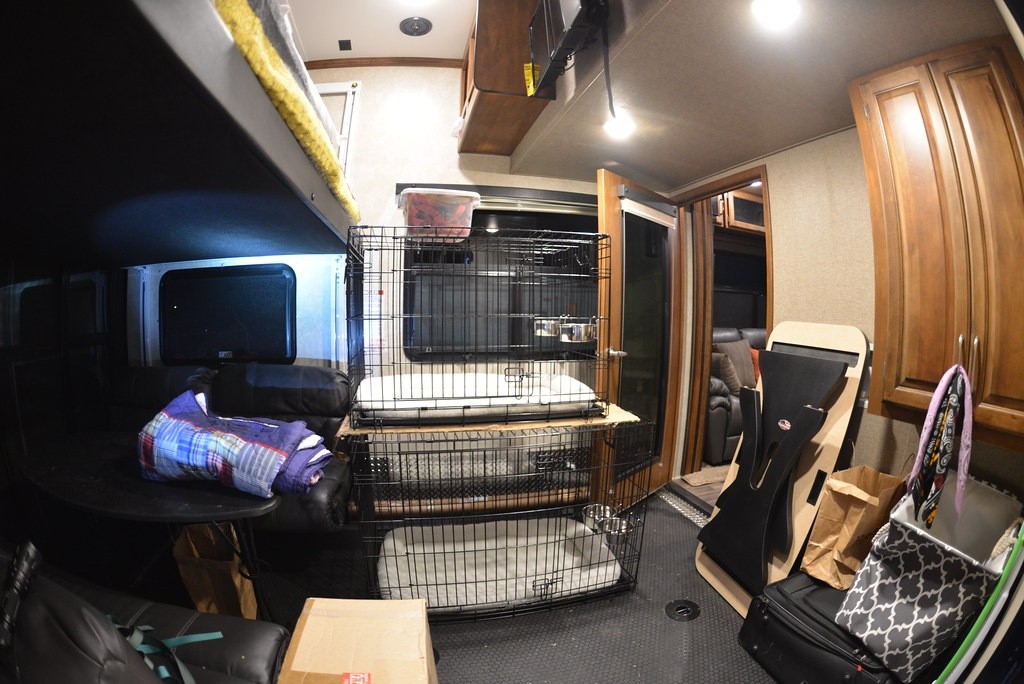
[844,32,1024,433]
[457,1,558,157]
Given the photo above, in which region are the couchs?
[0,536,291,684]
[108,360,362,546]
[702,327,767,466]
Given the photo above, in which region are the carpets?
[681,464,731,488]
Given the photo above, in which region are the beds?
[0,0,371,261]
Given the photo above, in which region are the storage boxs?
[398,188,480,246]
[276,597,439,684]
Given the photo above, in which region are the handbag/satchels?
[173,521,258,619]
[800,442,917,591]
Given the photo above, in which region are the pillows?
[713,338,757,389]
[749,346,760,383]
[710,352,743,396]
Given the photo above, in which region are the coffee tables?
[19,428,281,627]
